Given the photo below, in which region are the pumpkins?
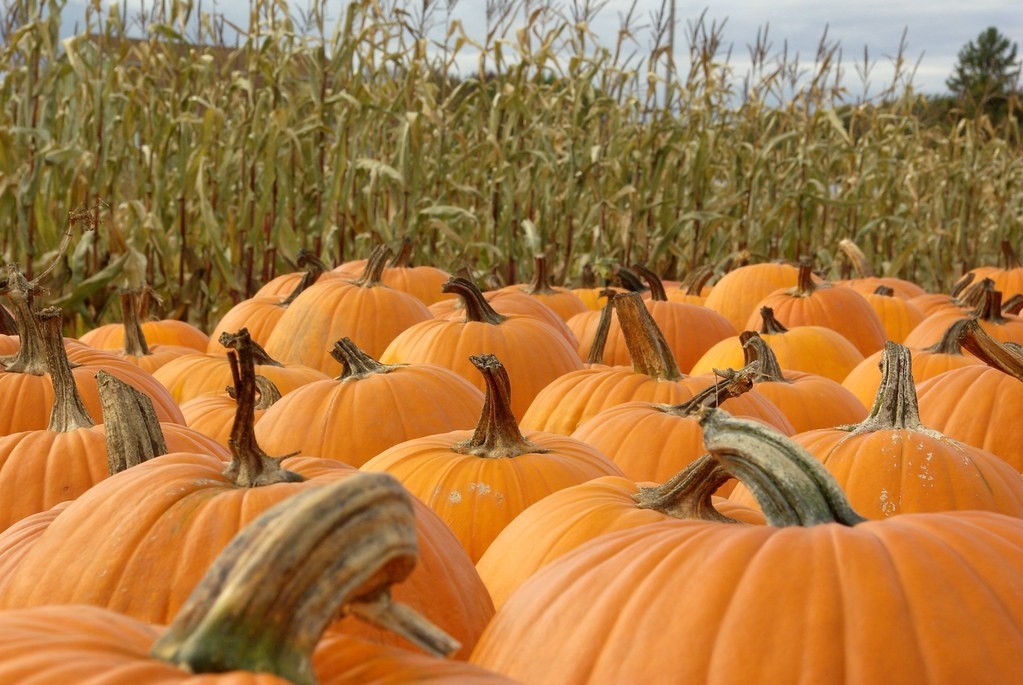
[0,220,1023,685]
